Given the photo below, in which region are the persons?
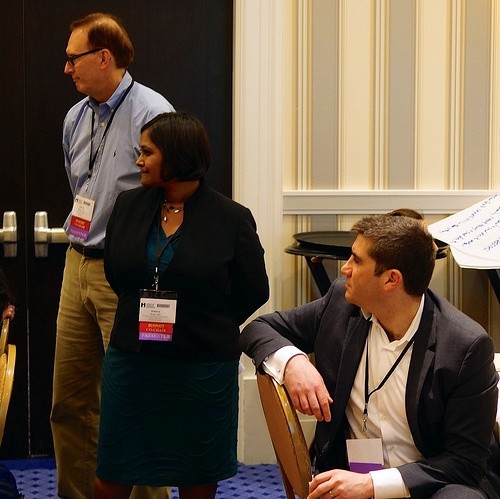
[241,216,500,499]
[93,111,271,499]
[50,13,176,499]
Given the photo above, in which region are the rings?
[329,491,334,497]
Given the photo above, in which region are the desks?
[284,231,449,297]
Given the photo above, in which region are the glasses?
[66,48,111,67]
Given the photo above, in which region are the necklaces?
[163,204,183,222]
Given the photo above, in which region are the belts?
[71,242,104,258]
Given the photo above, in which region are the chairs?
[257,371,312,499]
